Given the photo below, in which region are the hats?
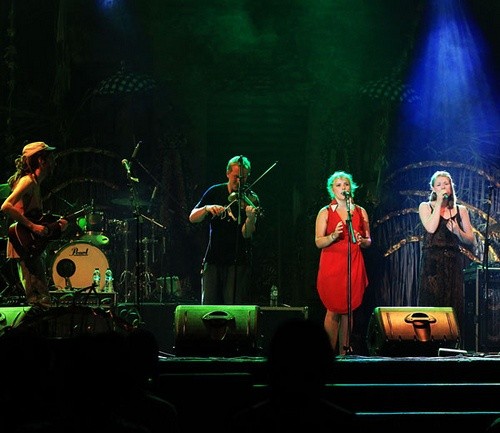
[22,141,56,157]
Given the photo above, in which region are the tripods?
[113,175,167,304]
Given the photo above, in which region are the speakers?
[175,305,261,357]
[366,306,461,358]
[0,306,34,335]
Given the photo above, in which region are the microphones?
[238,156,243,165]
[343,191,350,197]
[443,194,449,199]
[121,159,130,175]
[131,141,141,158]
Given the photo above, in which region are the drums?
[68,210,113,247]
[49,240,112,292]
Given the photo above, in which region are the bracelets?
[205,207,206,211]
[331,233,334,239]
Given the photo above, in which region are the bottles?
[104,267,114,294]
[93,267,101,294]
[270,284,277,308]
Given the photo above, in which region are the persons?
[315,171,371,355]
[188,156,260,304]
[418,170,474,346]
[0,142,68,303]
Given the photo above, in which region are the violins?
[228,187,265,220]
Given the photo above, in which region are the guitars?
[7,203,96,260]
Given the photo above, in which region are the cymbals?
[133,238,159,246]
[109,196,156,207]
